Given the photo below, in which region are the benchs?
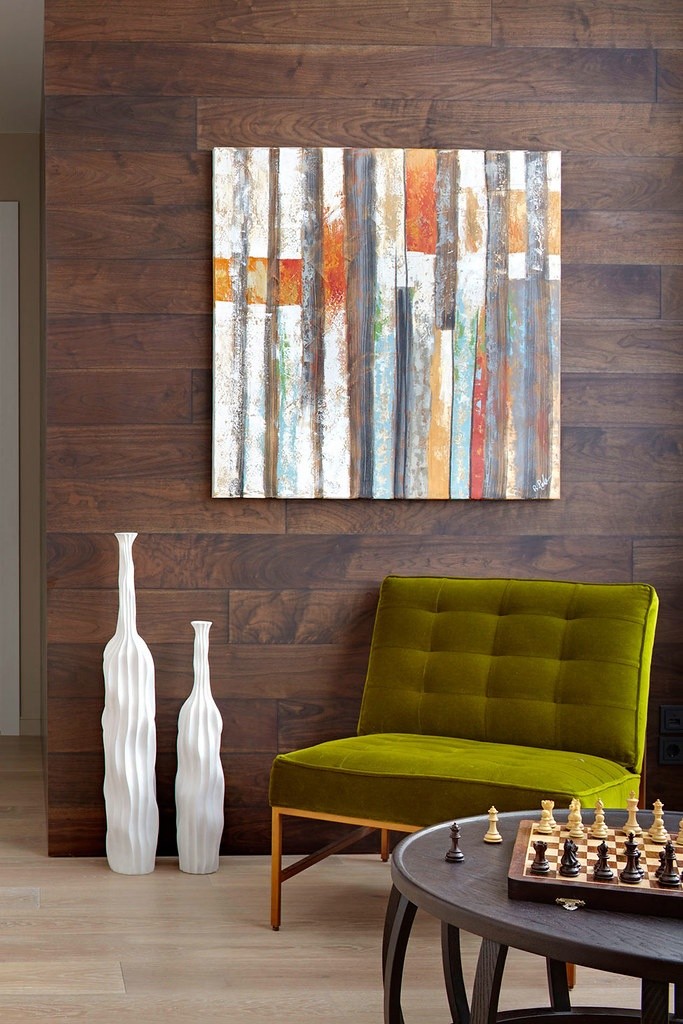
[268,574,661,931]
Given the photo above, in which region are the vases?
[103,533,160,875]
[174,619,226,875]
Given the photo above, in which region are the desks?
[384,806,683,1024]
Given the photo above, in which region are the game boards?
[507,818,683,921]
